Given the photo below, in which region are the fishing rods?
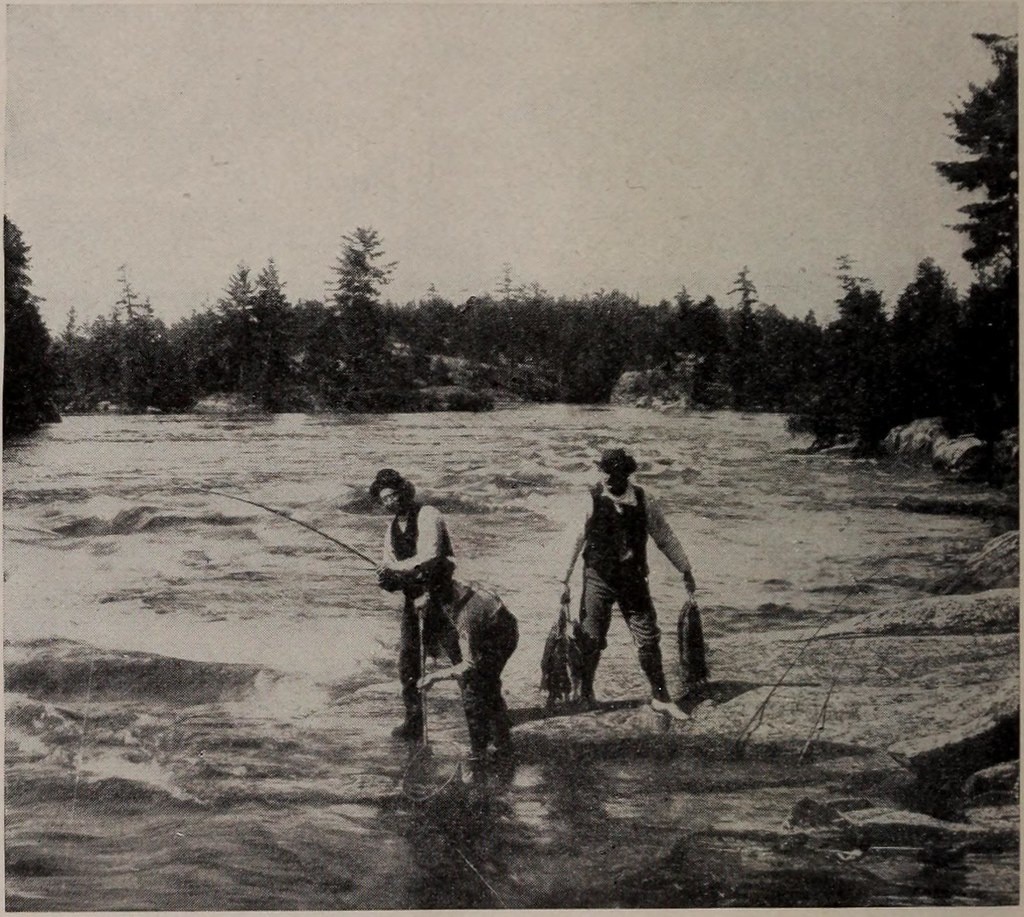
[118,485,381,573]
[710,556,896,759]
[798,585,879,763]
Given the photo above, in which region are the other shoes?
[651,698,692,720]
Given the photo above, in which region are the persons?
[560,448,697,722]
[368,468,520,766]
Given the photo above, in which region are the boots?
[392,694,423,737]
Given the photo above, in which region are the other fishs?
[675,600,713,693]
[541,606,587,705]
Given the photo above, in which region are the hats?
[370,468,415,500]
[592,449,637,475]
[416,556,456,582]
